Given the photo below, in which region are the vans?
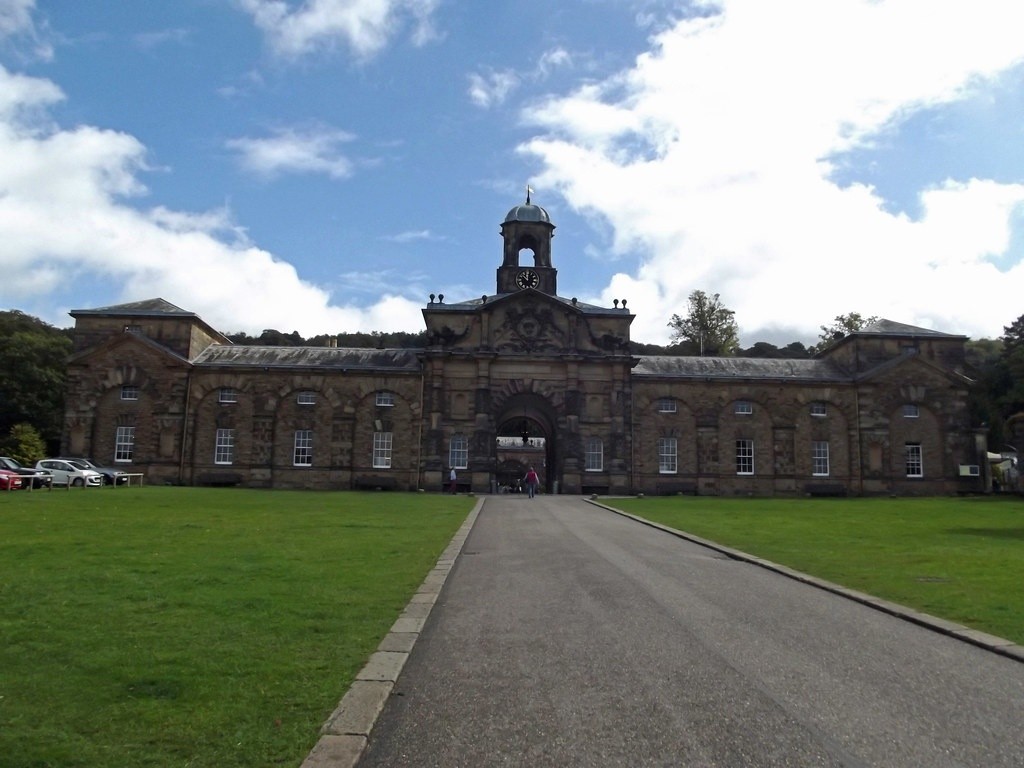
[35,459,104,488]
[57,457,128,485]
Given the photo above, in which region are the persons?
[523,467,540,498]
[991,477,1001,491]
[500,480,519,493]
[449,466,457,494]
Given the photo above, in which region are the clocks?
[516,268,539,289]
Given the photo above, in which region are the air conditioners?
[957,464,979,476]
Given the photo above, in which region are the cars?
[0,469,25,491]
[0,457,53,489]
